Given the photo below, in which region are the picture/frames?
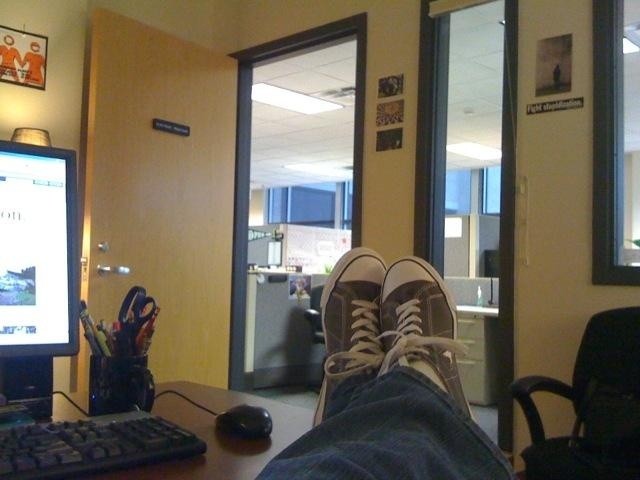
[0,26,48,90]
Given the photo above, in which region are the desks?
[37,379,513,480]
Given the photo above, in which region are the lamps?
[10,127,51,147]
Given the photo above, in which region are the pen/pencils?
[136,306,160,356]
[79,300,120,357]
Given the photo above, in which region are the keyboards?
[0,409,208,480]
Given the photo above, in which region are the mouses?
[216,404,273,439]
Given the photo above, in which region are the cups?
[87,350,156,416]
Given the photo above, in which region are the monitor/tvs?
[0,140,80,422]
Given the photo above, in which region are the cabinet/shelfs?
[453,305,499,406]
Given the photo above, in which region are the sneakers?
[312,247,475,430]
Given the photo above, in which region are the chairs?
[511,305,640,480]
[305,286,324,346]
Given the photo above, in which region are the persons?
[250,244,520,479]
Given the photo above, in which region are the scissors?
[119,286,156,355]
